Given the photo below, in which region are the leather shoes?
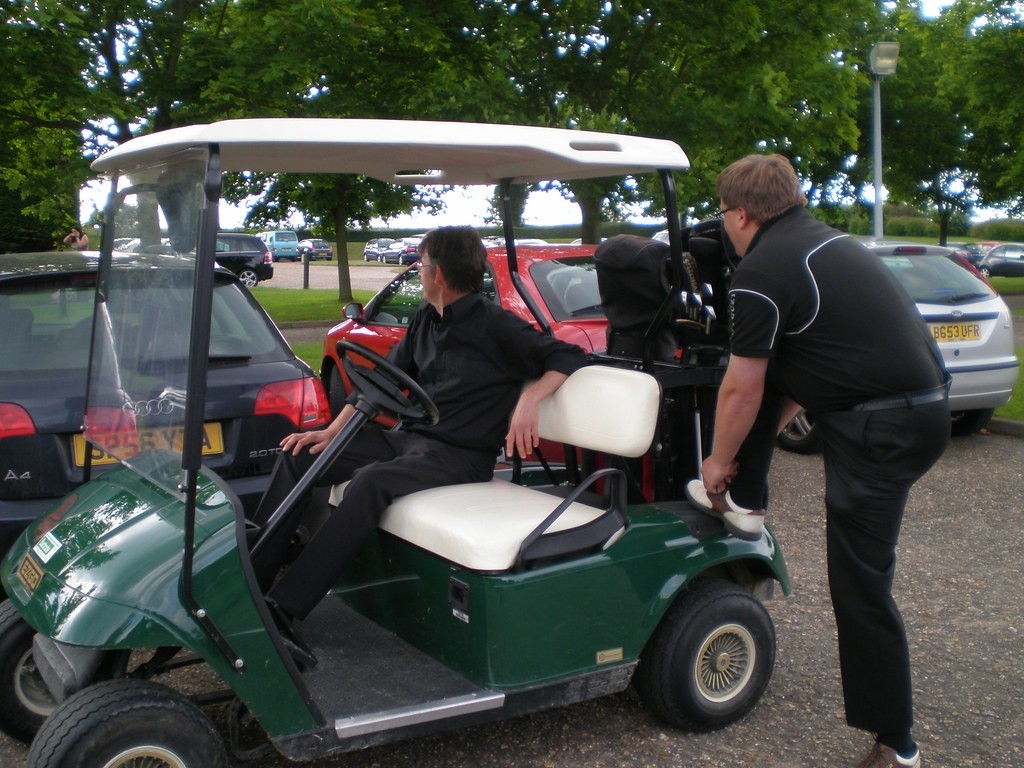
[856,739,921,768]
[686,478,765,541]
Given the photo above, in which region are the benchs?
[327,363,663,579]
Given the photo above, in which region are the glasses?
[714,205,735,222]
[416,259,431,272]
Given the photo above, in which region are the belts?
[851,366,954,413]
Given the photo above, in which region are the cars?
[650,229,671,246]
[979,243,1024,279]
[98,232,274,289]
[1,249,337,552]
[362,228,610,267]
[776,238,1020,454]
[932,241,1005,268]
[297,239,333,260]
[320,247,689,508]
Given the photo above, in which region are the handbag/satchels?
[673,220,771,461]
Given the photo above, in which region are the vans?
[255,231,298,262]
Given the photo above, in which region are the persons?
[139,287,261,358]
[685,155,968,763]
[232,202,587,669]
[64,218,97,250]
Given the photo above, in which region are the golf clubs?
[675,283,717,335]
